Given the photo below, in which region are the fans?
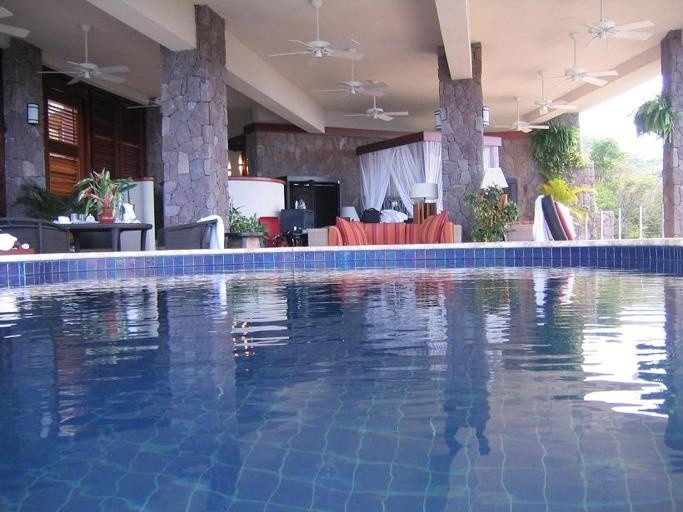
[268,0,409,122]
[35,24,131,86]
[491,1,655,134]
[124,96,161,110]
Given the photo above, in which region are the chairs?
[157,219,218,250]
[1,216,71,255]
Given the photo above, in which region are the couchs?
[305,221,463,246]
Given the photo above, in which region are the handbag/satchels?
[362,208,381,223]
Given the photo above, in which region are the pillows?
[336,217,368,246]
[418,210,449,245]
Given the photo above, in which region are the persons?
[69,242,76,253]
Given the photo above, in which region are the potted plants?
[73,167,138,224]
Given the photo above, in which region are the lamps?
[27,102,39,125]
[409,183,439,223]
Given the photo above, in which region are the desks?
[56,222,153,253]
